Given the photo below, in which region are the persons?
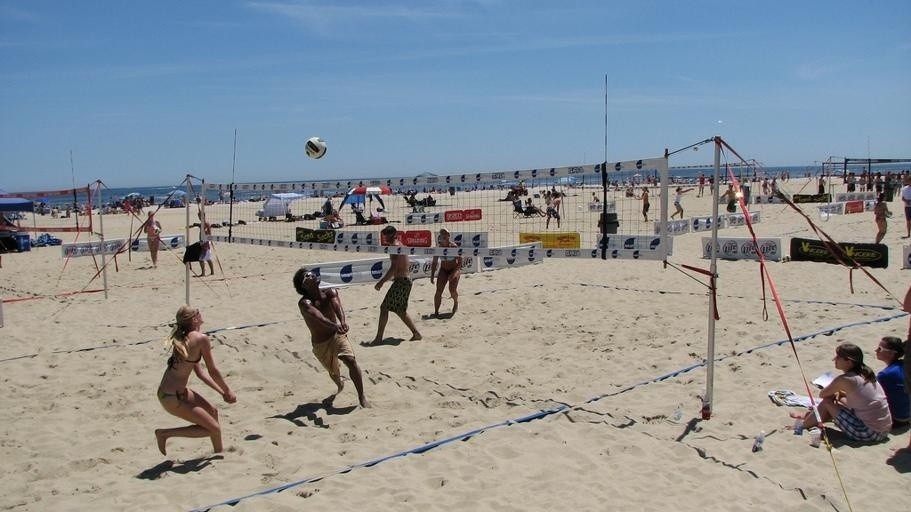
[372,225,426,344]
[294,267,373,409]
[186,210,216,277]
[875,336,911,430]
[430,227,462,315]
[155,305,239,456]
[902,288,911,397]
[35,168,911,246]
[804,342,893,444]
[144,210,162,265]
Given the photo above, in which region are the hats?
[381,226,395,234]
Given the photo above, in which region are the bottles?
[794,416,803,435]
[752,431,766,452]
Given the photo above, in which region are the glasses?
[302,272,311,284]
[876,345,897,353]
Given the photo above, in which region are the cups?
[809,430,821,448]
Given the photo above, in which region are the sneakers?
[808,427,822,447]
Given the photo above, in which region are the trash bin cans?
[598,213,620,234]
[16,235,30,252]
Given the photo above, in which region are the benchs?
[510,204,547,217]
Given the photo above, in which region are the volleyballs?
[693,147,698,151]
[305,136,326,159]
[153,227,160,234]
[578,206,583,211]
[886,211,892,218]
